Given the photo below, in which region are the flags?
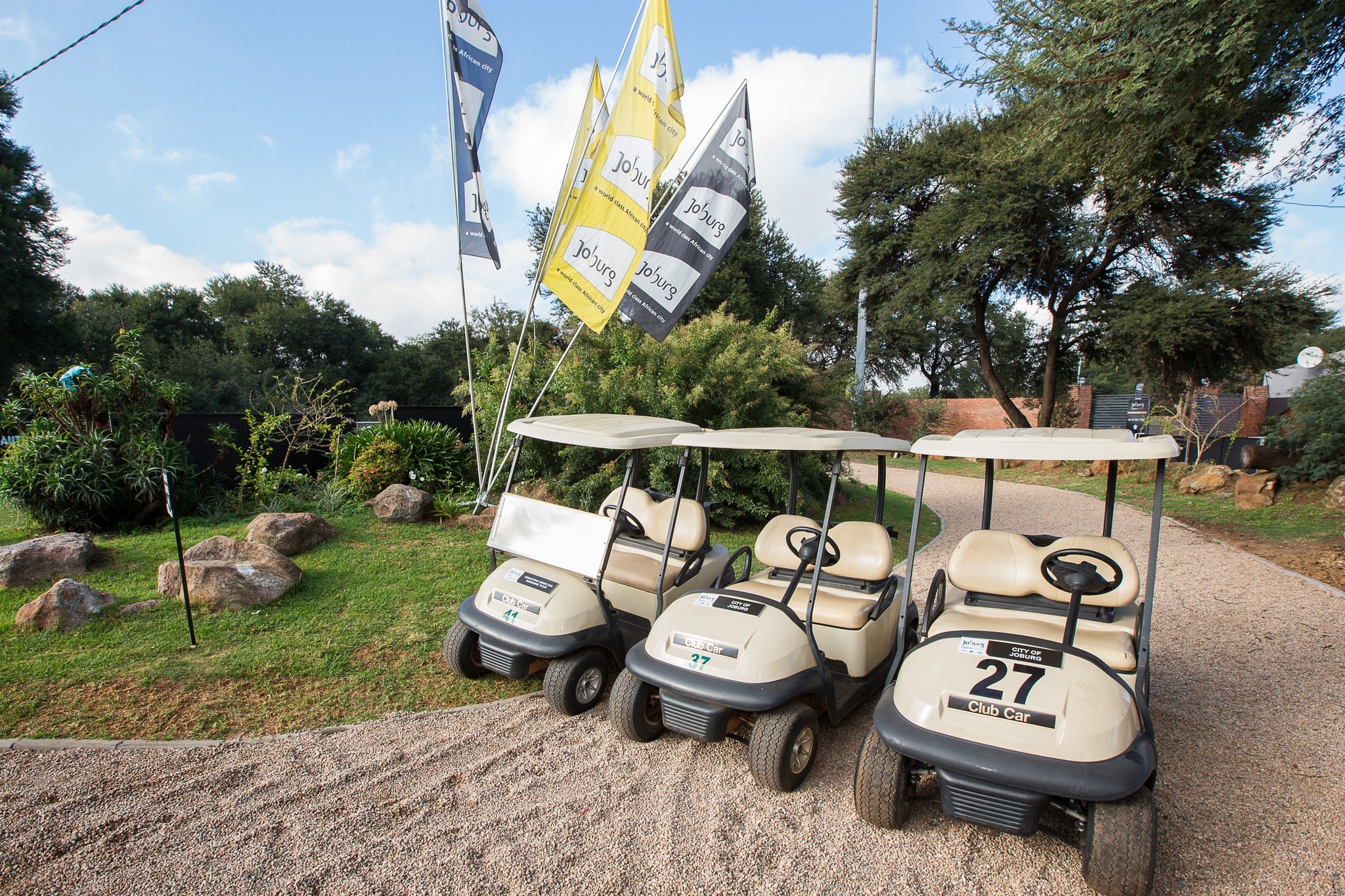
[537,0,687,336]
[612,82,758,343]
[440,0,502,270]
